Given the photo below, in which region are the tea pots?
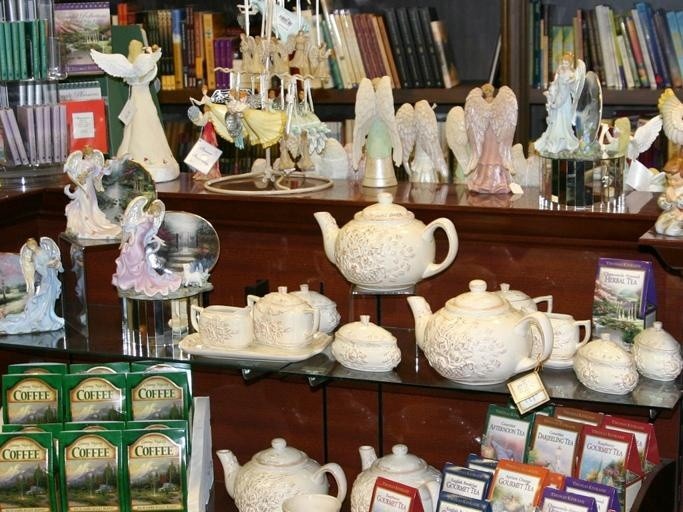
[314,192,461,291]
[406,279,555,386]
[349,444,441,511]
[217,436,348,512]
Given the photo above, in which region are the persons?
[59,144,123,241]
[0,236,65,339]
[110,201,182,296]
[652,154,683,238]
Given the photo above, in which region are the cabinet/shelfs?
[0,167,682,512]
[0,0,73,185]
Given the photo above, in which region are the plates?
[177,333,331,365]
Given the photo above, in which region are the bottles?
[331,313,404,372]
[189,284,337,346]
[572,321,682,393]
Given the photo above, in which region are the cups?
[537,310,592,368]
[284,495,344,511]
